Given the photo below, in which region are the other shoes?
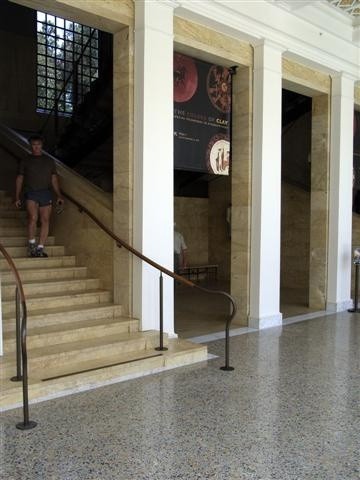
[38,248,49,258]
[27,242,37,256]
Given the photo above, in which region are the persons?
[14,135,65,258]
[173,222,188,286]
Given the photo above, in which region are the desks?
[177,264,218,282]
